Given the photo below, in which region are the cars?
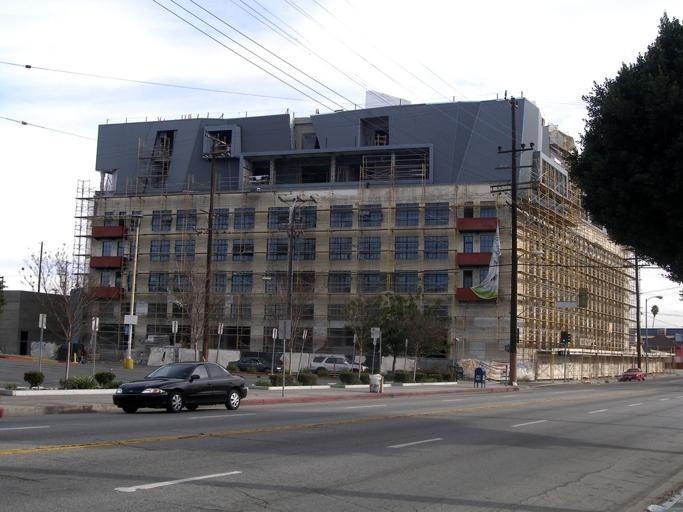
[623,366,644,382]
[227,356,283,376]
[112,360,248,414]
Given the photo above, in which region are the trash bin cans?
[369,374,382,393]
[474,367,485,388]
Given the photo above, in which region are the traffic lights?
[567,331,571,344]
[560,331,566,344]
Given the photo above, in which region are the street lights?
[644,294,664,376]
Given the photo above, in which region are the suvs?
[311,354,369,378]
